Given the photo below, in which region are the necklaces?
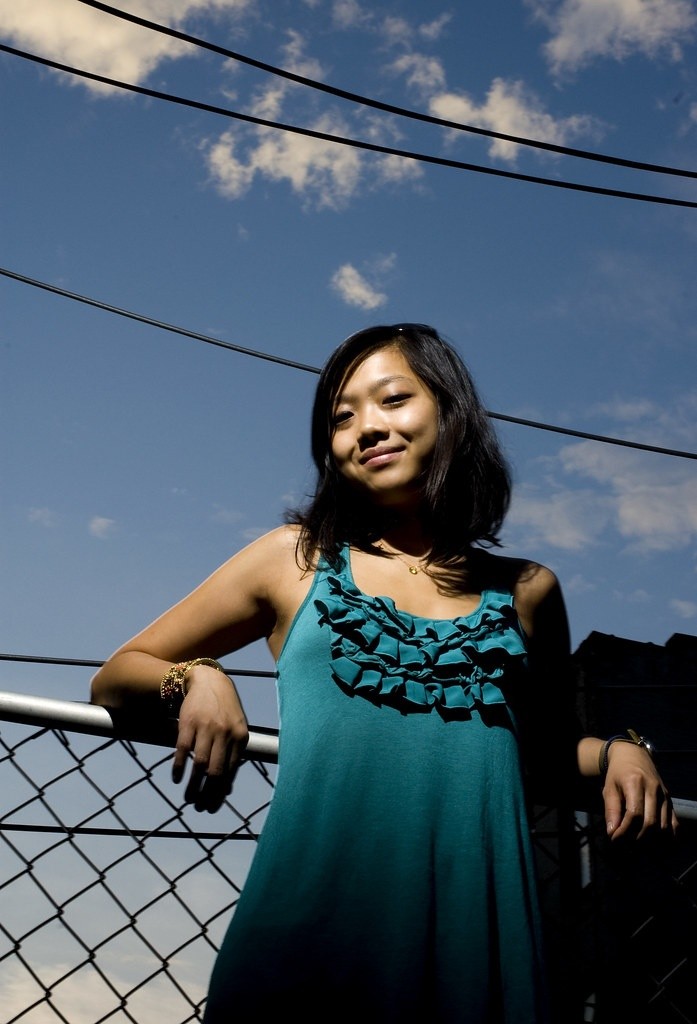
[377,536,429,576]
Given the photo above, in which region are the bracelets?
[603,734,623,773]
[159,658,223,717]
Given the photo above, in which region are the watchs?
[597,728,652,777]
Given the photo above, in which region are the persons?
[90,324,684,1024]
